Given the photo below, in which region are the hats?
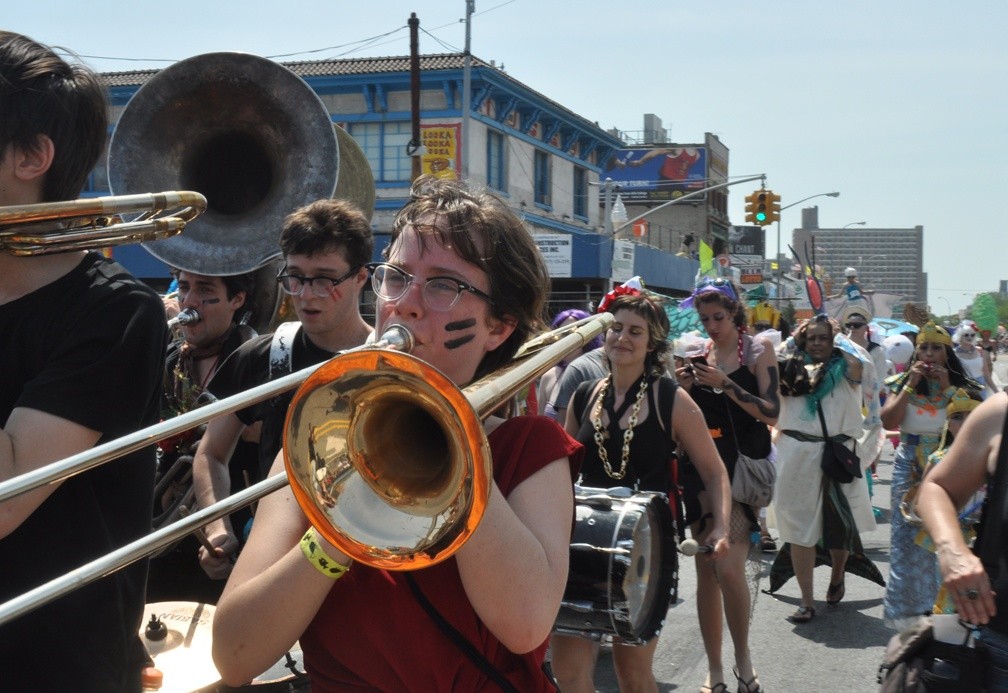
[844,267,857,277]
[843,305,872,324]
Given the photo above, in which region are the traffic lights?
[746,189,781,226]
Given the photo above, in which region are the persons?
[0,31,171,693]
[160,170,1008,693]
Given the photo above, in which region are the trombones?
[1,312,618,625]
[1,190,206,257]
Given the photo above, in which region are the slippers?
[700,683,727,693]
[792,607,816,622]
[732,665,764,693]
[828,581,845,604]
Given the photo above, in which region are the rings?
[967,585,979,600]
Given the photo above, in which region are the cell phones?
[691,355,708,371]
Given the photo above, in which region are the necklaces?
[590,367,657,479]
[711,344,725,370]
[198,356,219,391]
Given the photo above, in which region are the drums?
[551,484,679,647]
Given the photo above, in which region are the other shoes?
[760,532,777,552]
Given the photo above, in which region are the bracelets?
[902,384,914,393]
[299,524,352,579]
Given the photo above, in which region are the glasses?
[277,264,360,297]
[844,322,866,329]
[963,333,976,337]
[364,262,497,313]
[848,276,856,278]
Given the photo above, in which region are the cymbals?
[137,600,225,693]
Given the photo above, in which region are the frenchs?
[108,53,376,561]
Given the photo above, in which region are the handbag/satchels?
[731,455,776,508]
[821,441,863,483]
[778,358,826,397]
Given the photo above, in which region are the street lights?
[839,220,866,287]
[776,190,840,312]
